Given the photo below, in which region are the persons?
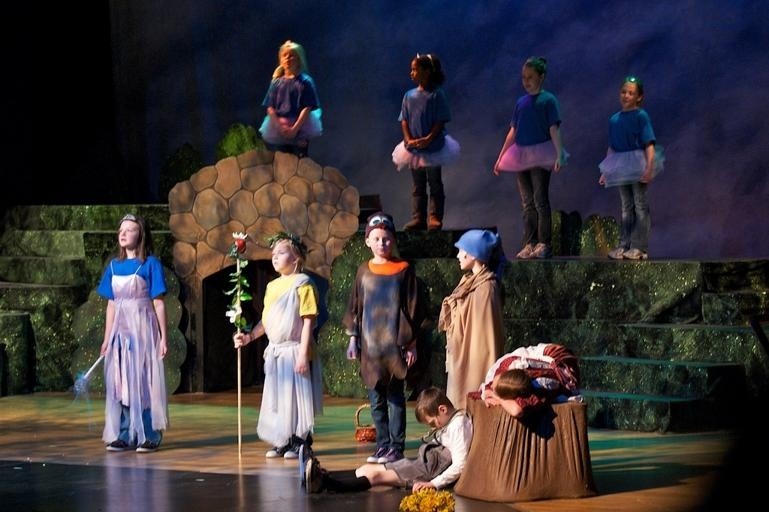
[233,232,319,459]
[441,229,508,409]
[298,387,474,493]
[260,40,323,157]
[492,56,569,261]
[343,211,421,462]
[391,53,458,232]
[599,76,663,261]
[480,341,575,422]
[96,212,169,453]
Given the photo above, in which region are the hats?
[365,213,396,235]
[454,229,502,263]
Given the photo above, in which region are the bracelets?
[249,332,255,342]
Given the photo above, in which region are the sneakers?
[402,214,424,230]
[606,248,626,262]
[299,444,312,487]
[136,442,160,454]
[368,447,404,464]
[306,460,324,494]
[285,450,301,459]
[622,248,649,261]
[515,243,533,259]
[424,215,444,229]
[105,440,127,452]
[529,242,553,259]
[266,447,283,459]
